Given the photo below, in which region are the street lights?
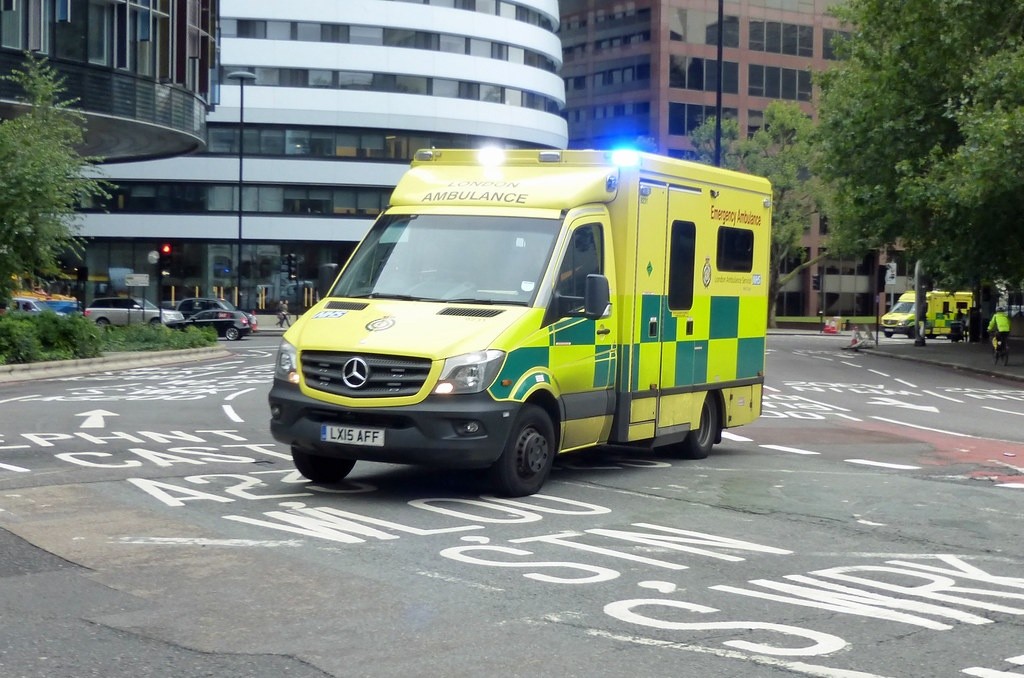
[228,70,257,311]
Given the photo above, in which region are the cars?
[5,297,82,320]
[165,309,251,341]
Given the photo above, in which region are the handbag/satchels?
[277,313,284,320]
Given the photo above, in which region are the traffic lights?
[288,252,298,281]
[78,266,89,282]
[159,242,173,268]
[812,274,822,293]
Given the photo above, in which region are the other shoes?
[995,349,999,354]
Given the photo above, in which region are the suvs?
[84,296,184,329]
[177,297,259,332]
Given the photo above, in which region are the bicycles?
[989,331,1010,366]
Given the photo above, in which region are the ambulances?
[269,149,774,500]
[881,291,976,339]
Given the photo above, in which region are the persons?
[275,299,290,328]
[988,310,1012,358]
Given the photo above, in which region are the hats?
[996,306,1005,312]
